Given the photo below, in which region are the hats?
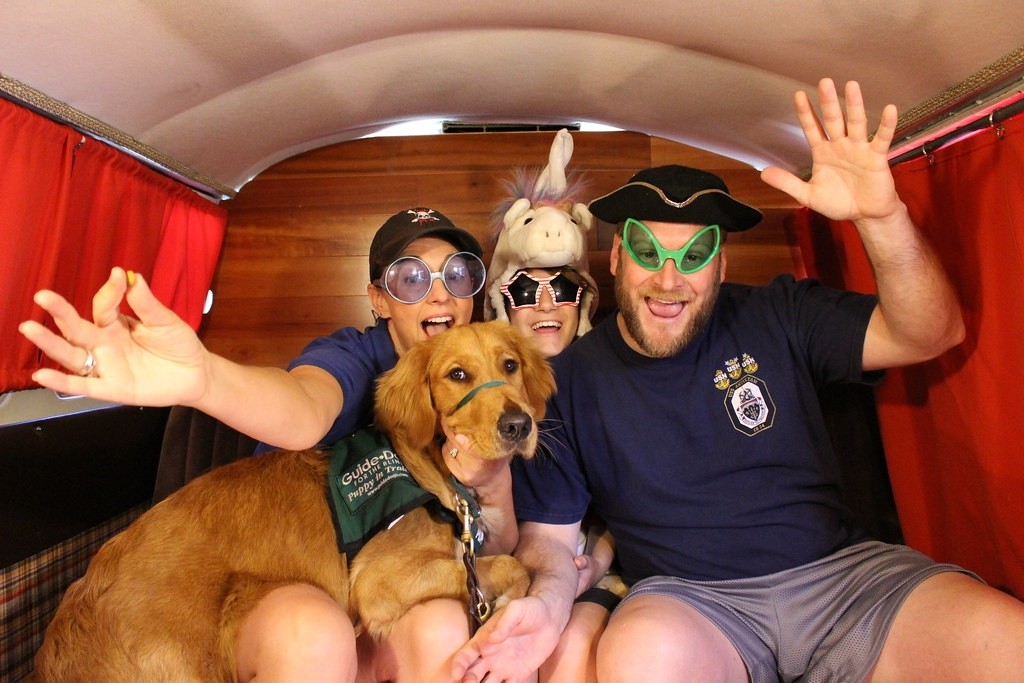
[481,127,600,336]
[369,206,483,284]
[587,164,764,233]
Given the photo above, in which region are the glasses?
[620,216,721,275]
[499,269,584,310]
[374,252,486,305]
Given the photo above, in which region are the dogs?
[34,320,557,683]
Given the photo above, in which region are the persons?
[483,127,633,683]
[18,204,520,683]
[450,75,1024,683]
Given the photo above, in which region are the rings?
[75,349,98,378]
